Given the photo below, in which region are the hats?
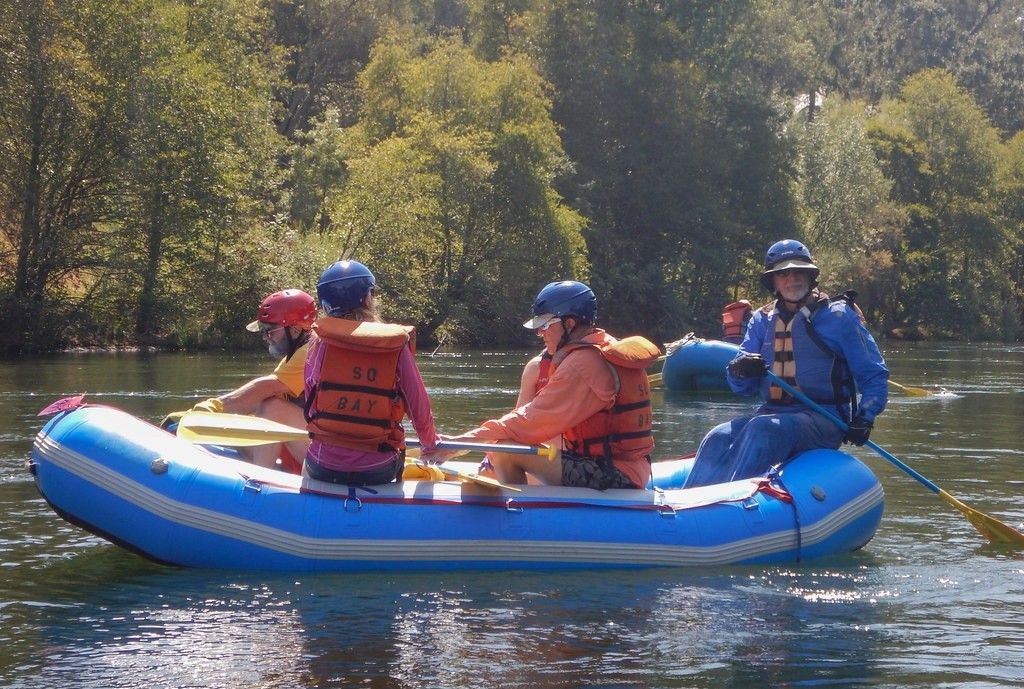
[522,312,556,329]
[760,256,823,294]
[245,320,282,332]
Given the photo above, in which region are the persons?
[300,260,444,488]
[420,280,654,491]
[159,288,319,475]
[679,238,889,488]
[480,346,562,485]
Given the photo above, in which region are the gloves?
[160,397,225,430]
[729,353,772,379]
[843,416,874,448]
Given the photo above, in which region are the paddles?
[648,372,664,387]
[175,408,560,464]
[765,368,1024,546]
[405,457,523,493]
[886,379,929,397]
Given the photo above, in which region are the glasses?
[540,316,571,330]
[369,288,375,297]
[262,327,285,337]
[773,267,807,276]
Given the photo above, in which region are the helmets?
[764,239,813,270]
[316,259,375,317]
[533,281,598,327]
[257,290,318,331]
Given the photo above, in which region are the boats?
[659,335,745,395]
[24,391,885,574]
